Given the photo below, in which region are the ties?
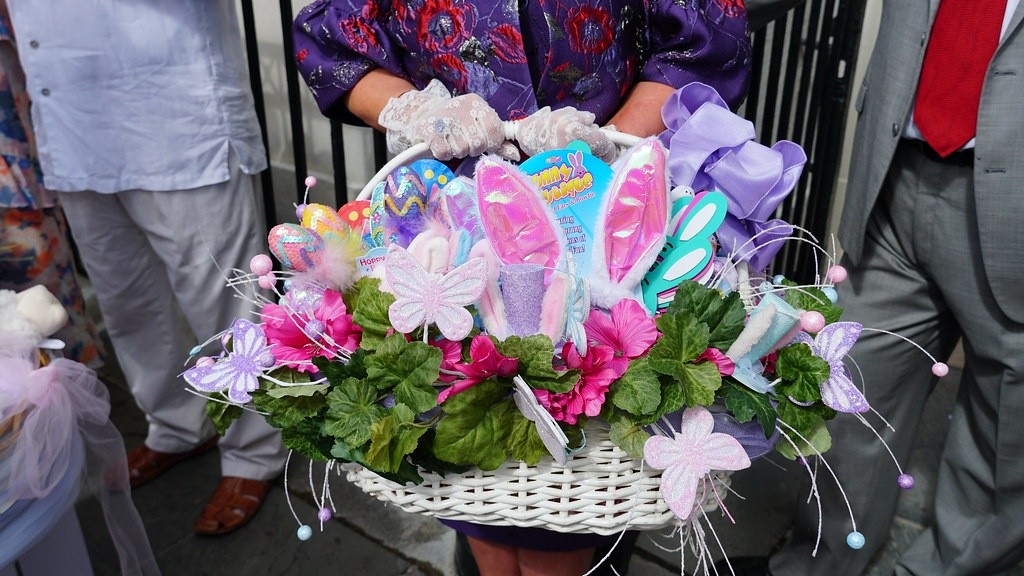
[914,1,1008,157]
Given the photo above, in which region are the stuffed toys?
[331,134,729,352]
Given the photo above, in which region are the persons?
[784,0,1023,574]
[292,3,757,575]
[6,1,279,537]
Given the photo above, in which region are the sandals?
[104,435,220,492]
[193,475,272,535]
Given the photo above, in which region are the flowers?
[168,73,951,576]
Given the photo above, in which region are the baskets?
[337,410,731,537]
